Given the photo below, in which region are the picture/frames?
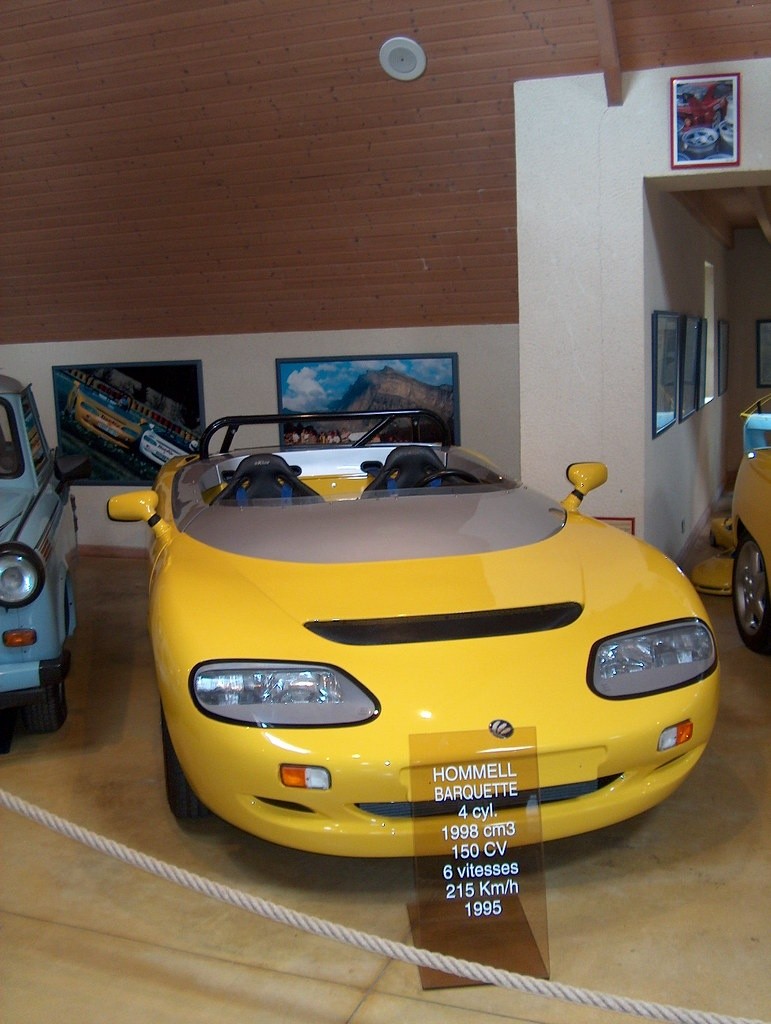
[718,320,729,396]
[652,309,709,438]
[51,359,208,486]
[756,318,771,388]
[670,72,741,169]
[275,351,461,454]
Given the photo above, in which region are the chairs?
[357,445,466,499]
[211,453,324,507]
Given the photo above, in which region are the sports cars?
[67,377,149,454]
[140,424,200,467]
[723,444,771,656]
[106,407,716,859]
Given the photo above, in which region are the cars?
[0,374,95,736]
[677,82,729,132]
